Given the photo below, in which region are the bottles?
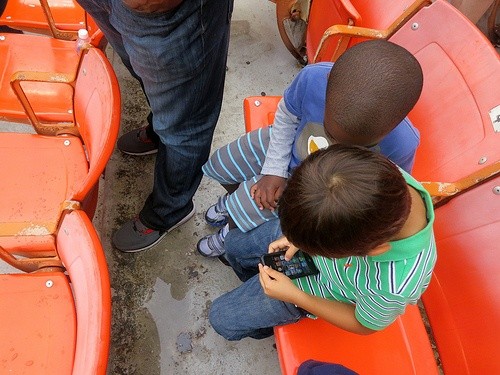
[75,29,90,56]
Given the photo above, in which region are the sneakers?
[196,223,229,258]
[205,193,231,226]
[117,125,158,155]
[113,201,196,252]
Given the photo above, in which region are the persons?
[76,0,234,253]
[197,40,424,258]
[209,144,437,341]
[296,359,359,375]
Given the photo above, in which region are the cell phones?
[260,249,319,280]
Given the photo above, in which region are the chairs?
[0,0,500,375]
